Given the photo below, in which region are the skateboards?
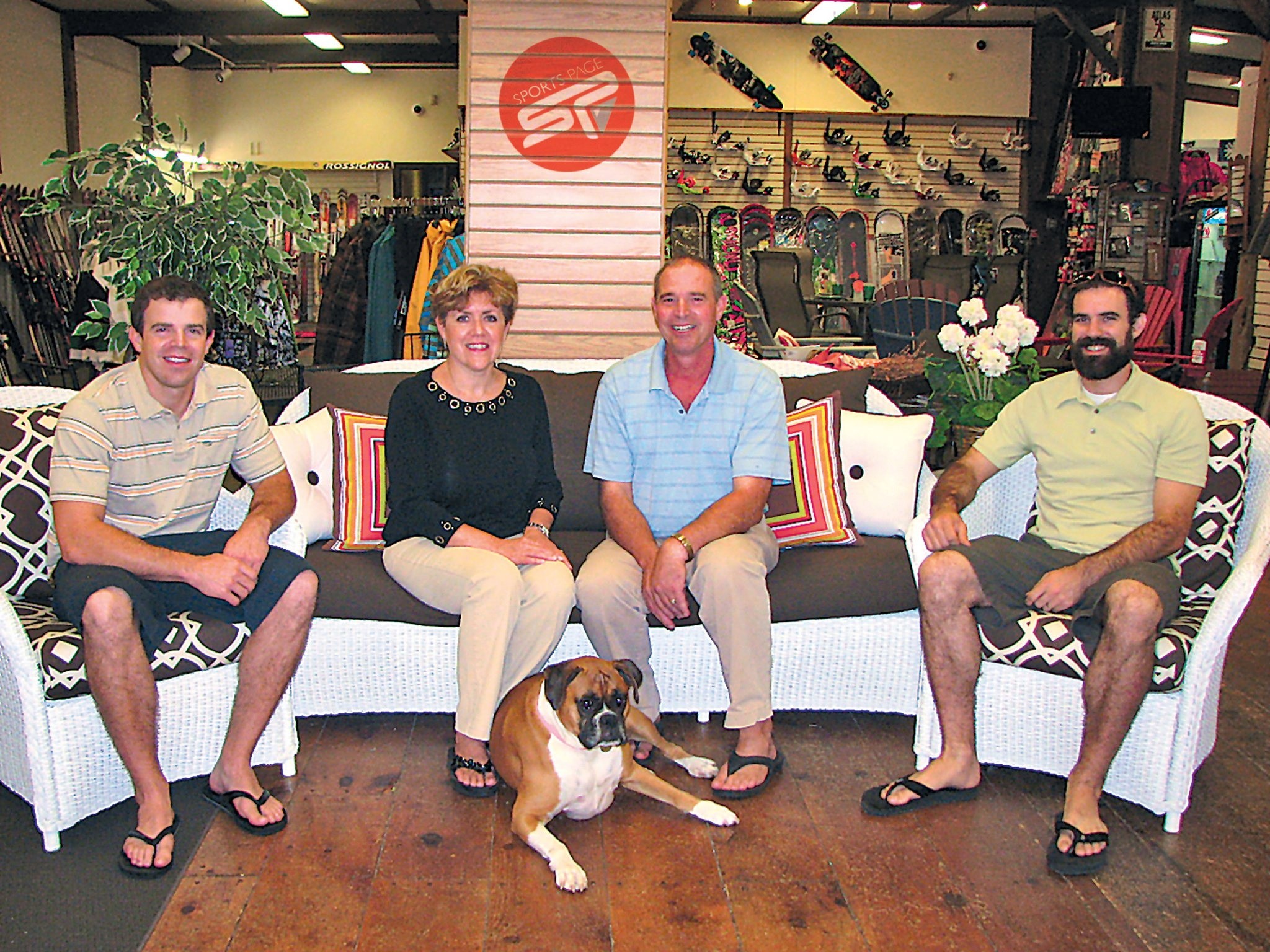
[687,31,784,110]
[809,31,894,113]
[668,197,1038,358]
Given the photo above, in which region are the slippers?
[710,745,784,797]
[860,775,983,818]
[628,713,668,767]
[117,814,181,879]
[1046,811,1109,874]
[445,741,501,798]
[202,780,290,835]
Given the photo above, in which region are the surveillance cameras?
[412,102,428,115]
[973,36,991,54]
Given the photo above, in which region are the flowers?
[913,294,1058,444]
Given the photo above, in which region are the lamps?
[298,32,345,53]
[341,59,371,76]
[213,59,232,82]
[800,0,855,30]
[261,0,312,20]
[172,33,192,61]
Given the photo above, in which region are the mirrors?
[1172,16,1266,379]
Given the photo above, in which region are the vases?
[945,425,988,460]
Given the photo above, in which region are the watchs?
[527,522,550,538]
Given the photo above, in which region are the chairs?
[0,383,306,858]
[740,222,1256,389]
[896,376,1269,836]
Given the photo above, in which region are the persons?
[1170,150,1230,212]
[46,276,322,879]
[575,254,790,799]
[379,263,575,799]
[858,266,1209,875]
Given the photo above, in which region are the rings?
[669,599,678,606]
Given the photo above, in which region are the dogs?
[489,656,740,894]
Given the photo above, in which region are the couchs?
[255,349,951,725]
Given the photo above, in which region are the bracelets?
[669,532,694,563]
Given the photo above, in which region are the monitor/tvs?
[1070,84,1152,139]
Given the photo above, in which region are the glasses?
[1068,268,1141,300]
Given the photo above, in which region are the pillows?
[0,400,81,602]
[778,362,876,425]
[741,390,863,552]
[1026,415,1259,588]
[793,394,938,541]
[315,398,398,555]
[258,403,350,554]
